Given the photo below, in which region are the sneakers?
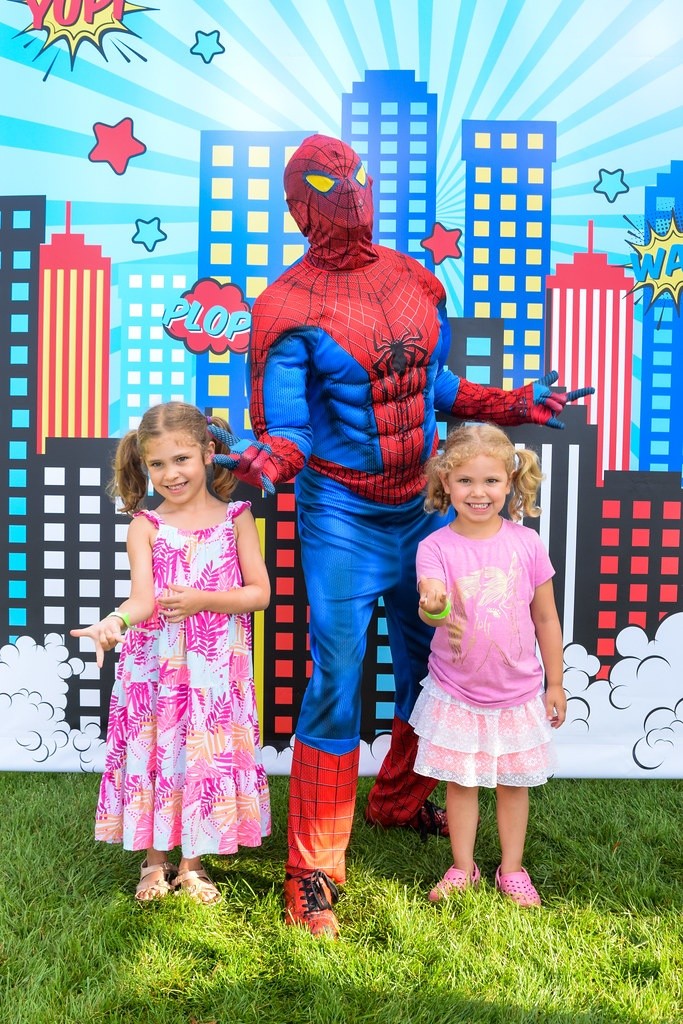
[283,870,341,938]
[406,798,481,843]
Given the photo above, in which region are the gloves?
[524,371,595,429]
[206,424,277,495]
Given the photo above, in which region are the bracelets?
[107,611,130,635]
[422,600,452,620]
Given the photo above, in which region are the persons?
[416,421,568,909]
[207,136,595,946]
[70,403,271,908]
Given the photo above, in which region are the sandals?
[136,858,177,899]
[428,862,480,901]
[172,861,221,903]
[496,864,540,907]
